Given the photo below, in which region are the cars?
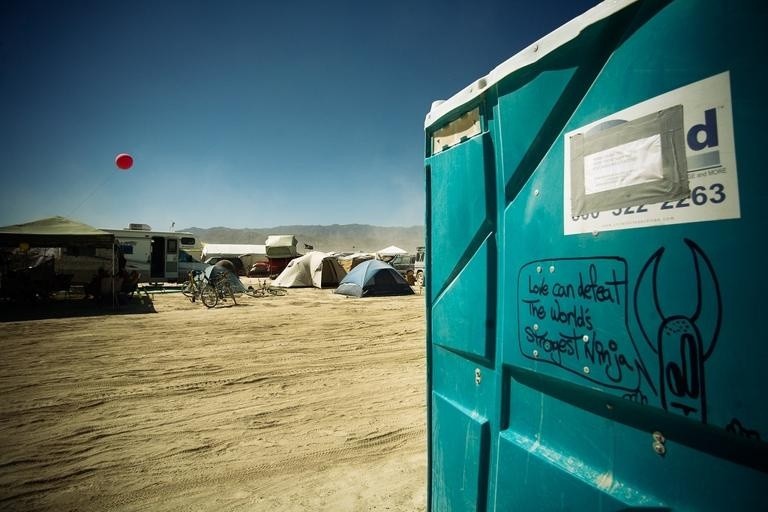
[248,267,265,276]
[375,247,425,286]
[203,257,244,275]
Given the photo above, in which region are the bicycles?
[245,280,287,297]
[182,270,236,308]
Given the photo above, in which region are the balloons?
[114,152,135,170]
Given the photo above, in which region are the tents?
[184,265,248,293]
[333,258,416,298]
[270,250,347,289]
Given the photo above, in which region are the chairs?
[51,273,73,297]
[88,271,141,306]
